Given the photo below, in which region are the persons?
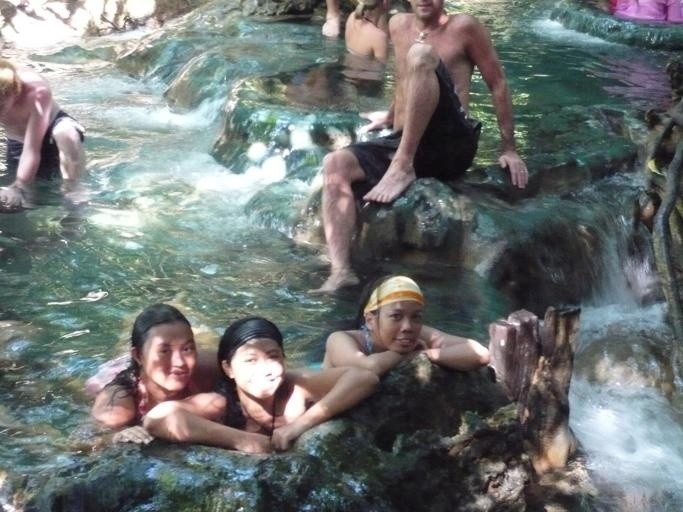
[308,1,531,297]
[140,315,382,456]
[0,54,90,210]
[318,1,341,36]
[608,0,683,24]
[344,1,390,65]
[89,302,220,448]
[320,272,493,378]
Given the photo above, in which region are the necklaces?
[414,31,427,44]
